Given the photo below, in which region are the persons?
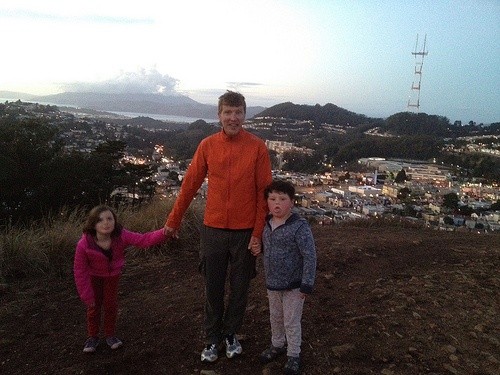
[248,179,317,375]
[73,204,179,352]
[164,91,272,362]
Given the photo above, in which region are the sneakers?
[224,335,242,358]
[107,335,123,349]
[83,338,99,353]
[201,339,223,363]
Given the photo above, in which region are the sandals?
[261,346,286,362]
[283,356,303,375]
